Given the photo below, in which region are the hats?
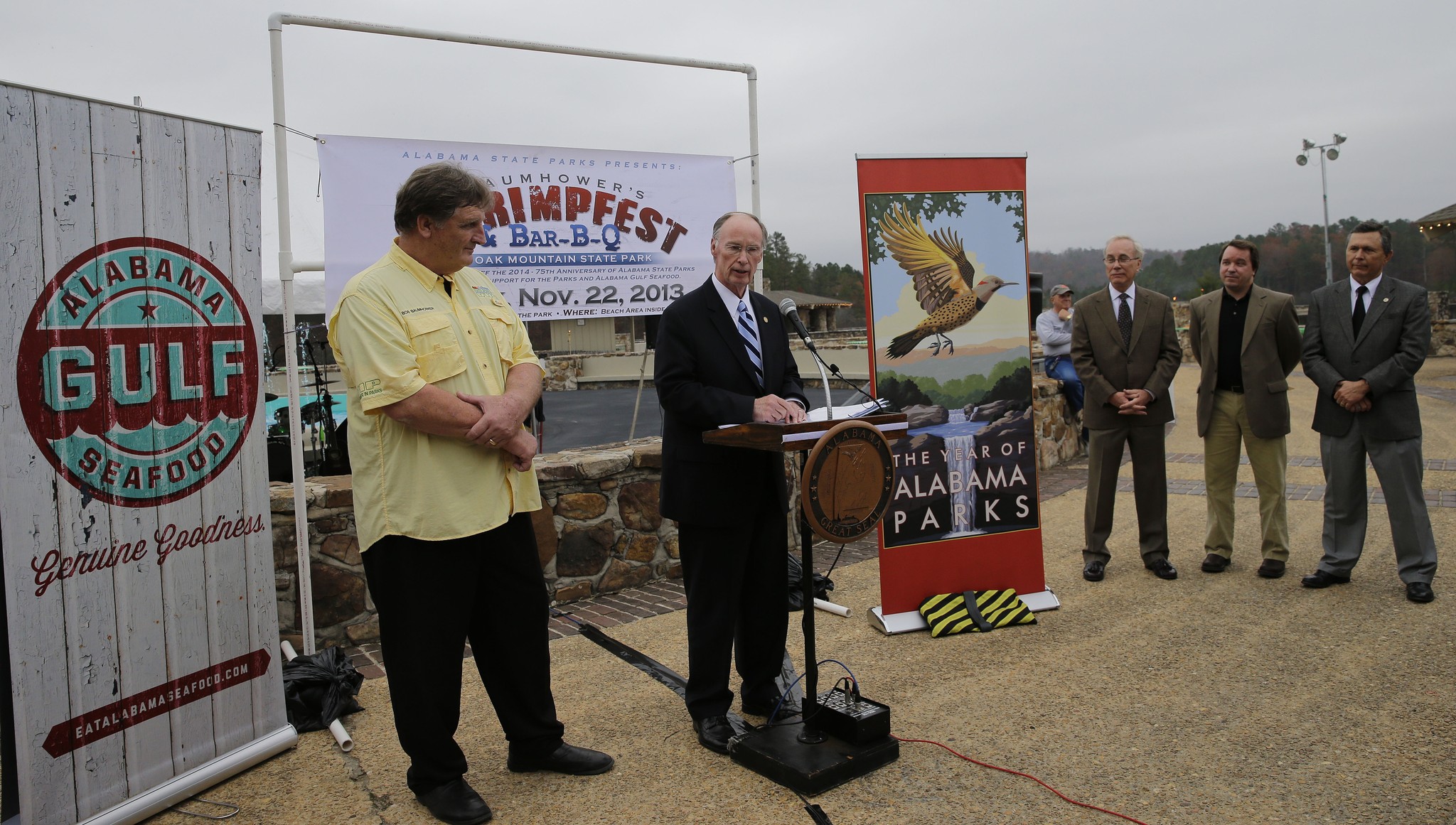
[1050,284,1074,298]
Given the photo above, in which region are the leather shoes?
[1083,560,1105,581]
[1301,569,1351,588]
[415,775,493,824]
[1201,554,1231,571]
[741,693,802,721]
[692,715,738,754]
[1258,558,1286,577]
[1145,558,1177,579]
[1407,582,1434,603]
[507,742,615,775]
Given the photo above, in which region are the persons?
[327,162,613,825]
[1299,221,1438,603]
[1036,284,1085,431]
[1070,235,1182,582]
[653,211,810,756]
[1189,240,1304,578]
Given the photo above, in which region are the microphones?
[779,298,817,353]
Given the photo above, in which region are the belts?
[1216,385,1244,394]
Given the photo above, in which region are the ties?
[737,300,764,391]
[1118,293,1132,352]
[1352,285,1369,339]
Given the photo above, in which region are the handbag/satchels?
[281,644,366,732]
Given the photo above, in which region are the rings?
[489,439,496,445]
[1356,406,1361,410]
[1341,402,1345,405]
[798,410,803,412]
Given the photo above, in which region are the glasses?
[1102,257,1140,265]
[715,237,763,256]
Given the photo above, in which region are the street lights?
[1294,133,1346,287]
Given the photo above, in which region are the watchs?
[1066,314,1072,321]
